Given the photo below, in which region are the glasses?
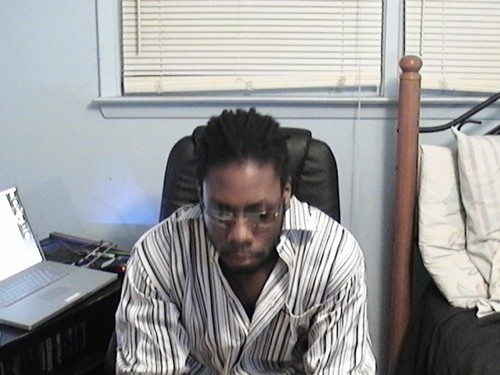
[197,186,283,232]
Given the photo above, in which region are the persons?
[114,107,377,375]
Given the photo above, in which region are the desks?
[0,231,131,375]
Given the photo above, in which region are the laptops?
[0,185,118,331]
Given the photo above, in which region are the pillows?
[450,125,500,319]
[417,143,490,310]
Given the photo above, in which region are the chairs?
[102,126,341,374]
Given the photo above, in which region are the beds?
[386,54,500,375]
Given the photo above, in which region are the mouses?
[101,256,129,274]
